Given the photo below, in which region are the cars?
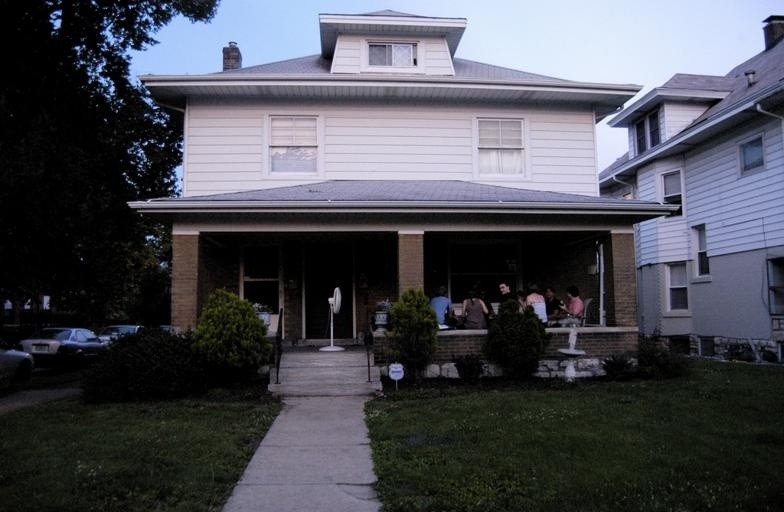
[0,323,168,395]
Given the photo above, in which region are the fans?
[319,287,346,352]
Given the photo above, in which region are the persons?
[457,281,584,328]
[427,286,457,330]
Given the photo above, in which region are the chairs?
[567,298,593,327]
